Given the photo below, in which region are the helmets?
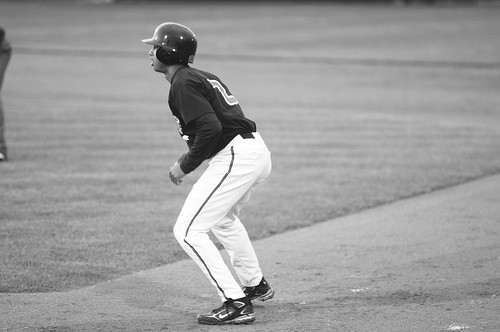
[140,22,197,64]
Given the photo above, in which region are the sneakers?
[196,297,255,323]
[240,277,275,300]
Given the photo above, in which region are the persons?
[141,20,274,325]
[0,23,14,162]
